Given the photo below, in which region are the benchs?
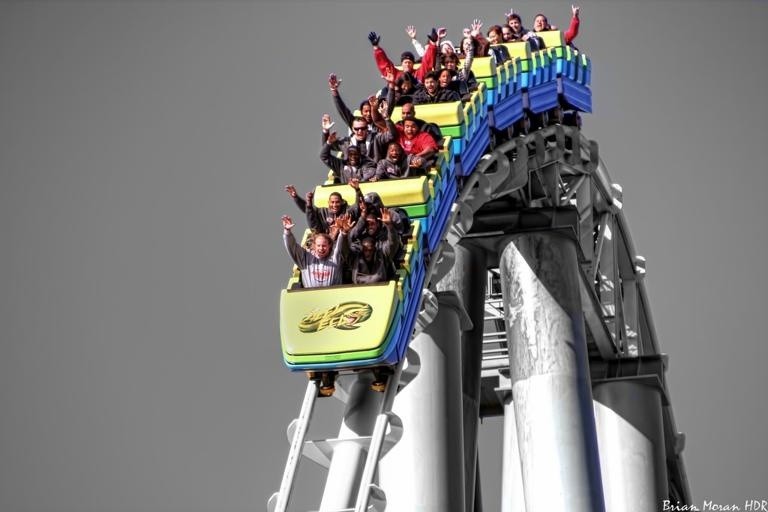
[288,28,590,319]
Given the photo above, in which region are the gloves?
[427,28,439,43]
[367,31,381,45]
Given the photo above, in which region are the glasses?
[353,127,368,130]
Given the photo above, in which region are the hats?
[400,51,417,63]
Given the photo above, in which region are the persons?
[280,4,582,293]
